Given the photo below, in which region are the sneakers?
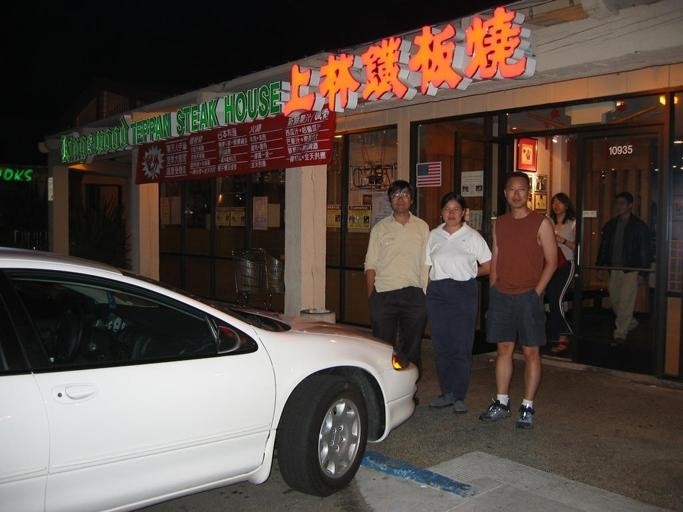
[550,345,568,352]
[515,404,536,429]
[478,399,513,423]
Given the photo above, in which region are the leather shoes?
[452,398,468,413]
[428,392,455,408]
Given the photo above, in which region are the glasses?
[390,191,411,200]
[442,206,462,212]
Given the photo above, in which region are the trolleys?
[229,247,284,311]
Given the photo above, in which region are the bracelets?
[562,239,566,244]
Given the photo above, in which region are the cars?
[0,247,420,511]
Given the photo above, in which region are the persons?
[594,192,650,342]
[424,191,491,413]
[362,180,431,378]
[547,192,576,353]
[478,170,558,430]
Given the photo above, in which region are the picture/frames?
[514,135,539,173]
[526,172,550,215]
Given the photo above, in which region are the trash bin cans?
[300,308,336,324]
